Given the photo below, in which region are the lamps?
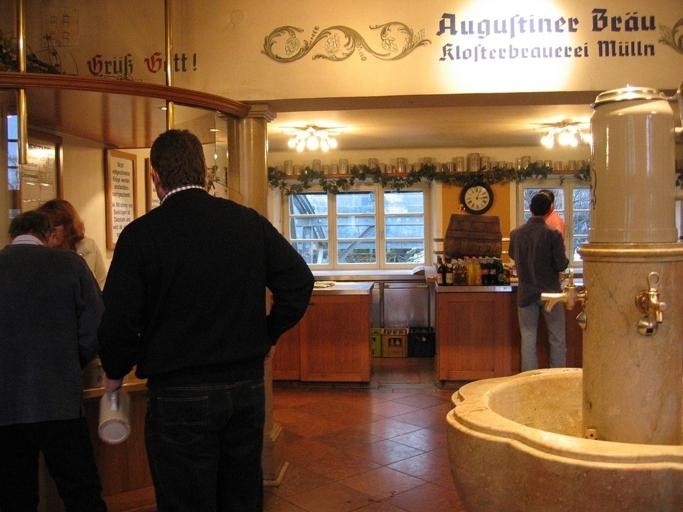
[287,122,339,154]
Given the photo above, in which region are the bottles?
[382,329,407,346]
[439,254,505,286]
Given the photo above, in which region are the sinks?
[445,368,683,512]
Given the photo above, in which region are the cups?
[275,158,396,174]
[98,386,131,444]
[396,153,587,172]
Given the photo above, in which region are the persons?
[0,210,108,512]
[508,192,568,372]
[539,189,566,244]
[38,198,108,291]
[97,130,315,512]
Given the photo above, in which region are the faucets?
[541,271,588,313]
[636,271,667,324]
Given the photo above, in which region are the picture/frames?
[106,148,138,252]
[145,157,161,214]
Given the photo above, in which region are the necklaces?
[160,185,205,205]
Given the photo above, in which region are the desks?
[269,280,376,382]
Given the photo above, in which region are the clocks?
[459,181,494,215]
